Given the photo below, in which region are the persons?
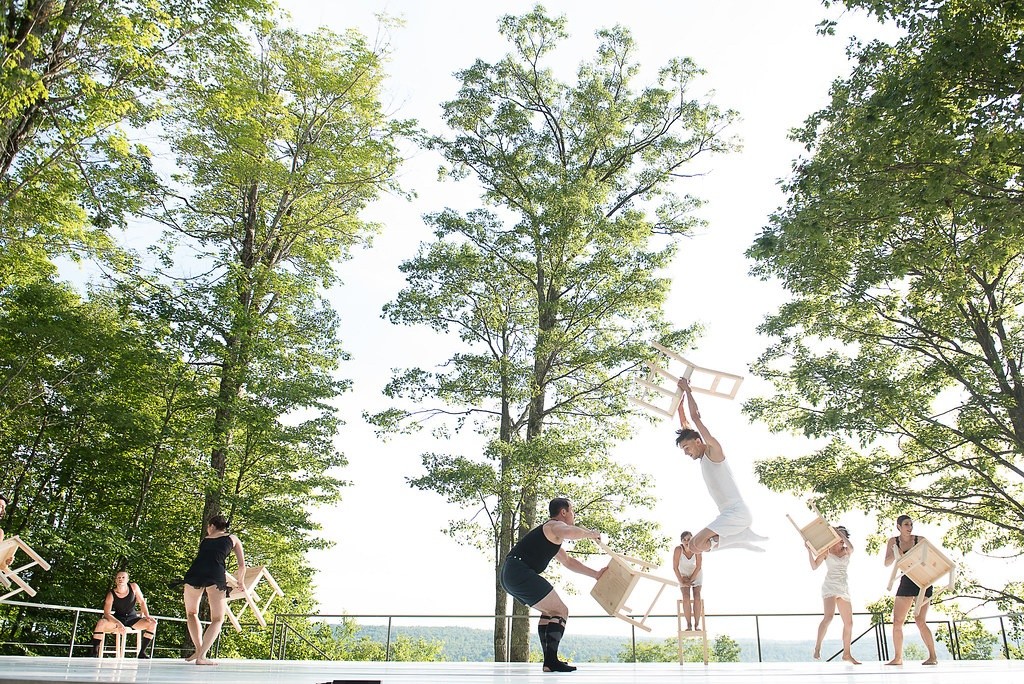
[0,493,15,565]
[500,498,609,672]
[183,515,247,665]
[92,570,157,659]
[673,531,702,631]
[884,515,938,665]
[804,526,862,665]
[675,376,769,555]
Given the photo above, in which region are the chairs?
[675,600,708,666]
[580,525,679,632]
[99,598,146,660]
[225,568,284,633]
[627,341,746,420]
[787,501,842,560]
[0,534,51,597]
[886,537,956,617]
